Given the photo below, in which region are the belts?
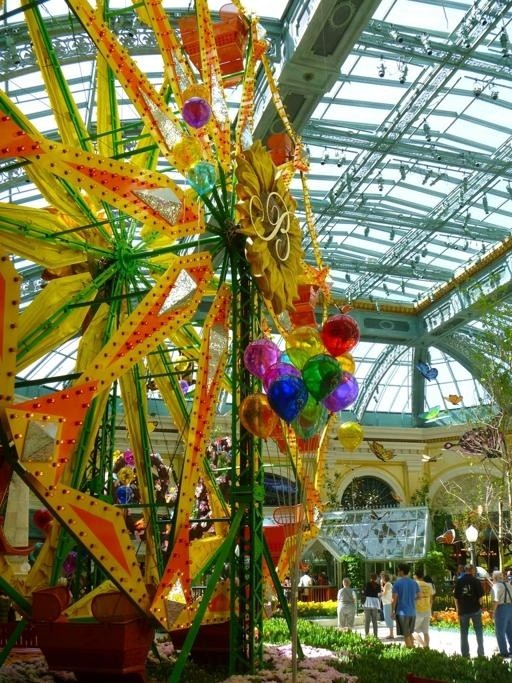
[498,601,510,608]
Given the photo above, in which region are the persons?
[452,563,485,657]
[297,568,313,600]
[423,572,437,593]
[413,569,433,648]
[283,577,292,603]
[362,572,382,637]
[492,570,512,657]
[337,577,357,631]
[378,569,395,638]
[391,562,420,648]
[507,568,512,584]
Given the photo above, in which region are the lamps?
[305,0,512,315]
[3,30,23,66]
[127,15,141,36]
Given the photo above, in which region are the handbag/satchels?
[360,582,368,604]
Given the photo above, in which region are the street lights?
[465,524,479,564]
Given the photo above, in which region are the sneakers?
[386,635,394,639]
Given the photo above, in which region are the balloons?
[235,313,360,440]
[172,136,201,172]
[336,419,365,452]
[123,448,136,464]
[174,355,189,372]
[33,508,52,530]
[118,467,134,484]
[179,380,187,394]
[185,162,217,196]
[117,486,135,504]
[30,542,44,560]
[182,95,212,128]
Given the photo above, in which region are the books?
[63,550,77,573]
